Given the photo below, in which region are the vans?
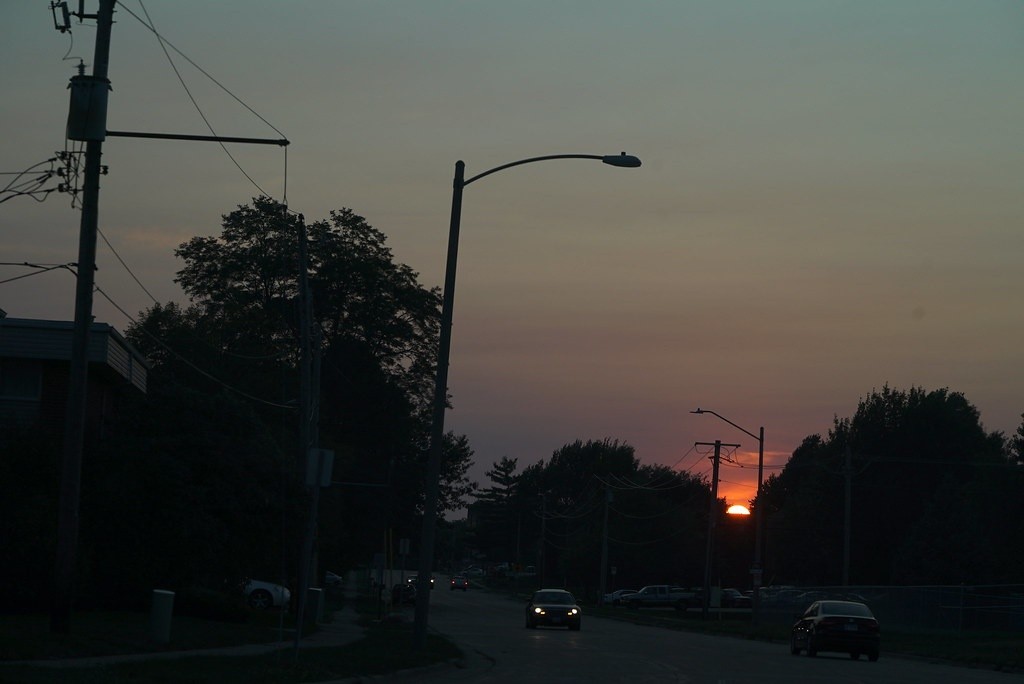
[392,584,415,605]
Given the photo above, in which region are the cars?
[603,589,636,601]
[527,589,582,631]
[792,600,881,660]
[451,576,467,590]
[461,565,482,576]
[430,575,436,587]
[409,574,417,584]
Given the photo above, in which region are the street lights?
[691,408,766,638]
[416,150,642,632]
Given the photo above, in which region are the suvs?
[720,589,751,608]
[622,585,678,607]
